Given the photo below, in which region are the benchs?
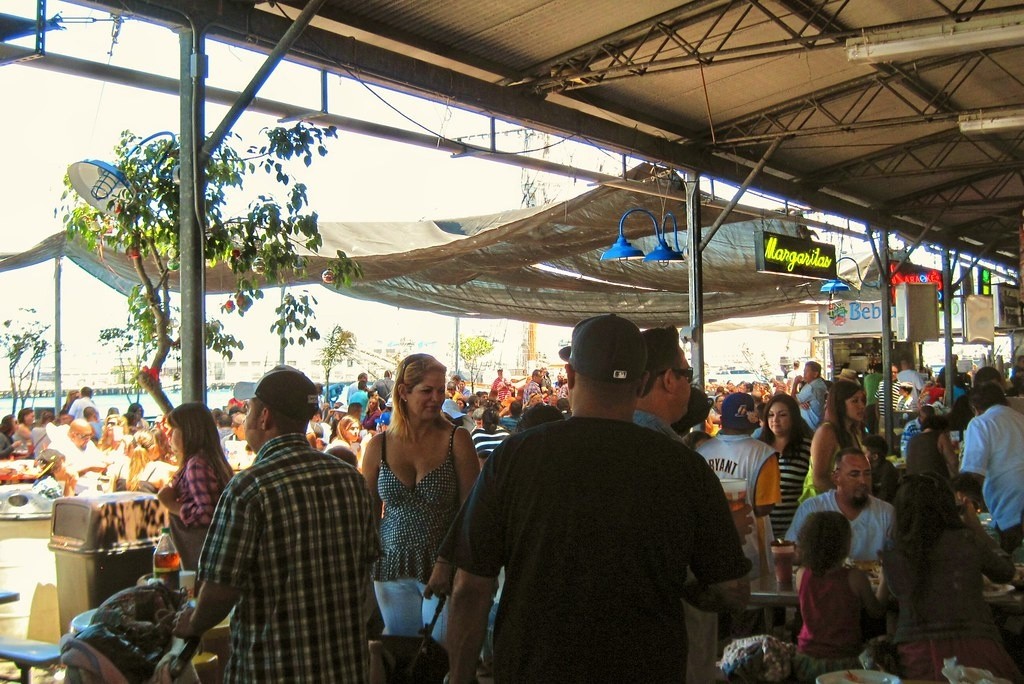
[0,635,62,684]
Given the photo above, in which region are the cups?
[720,478,747,513]
[770,540,796,584]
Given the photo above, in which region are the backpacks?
[75,581,190,681]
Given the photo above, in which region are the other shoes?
[772,604,786,627]
[791,612,803,643]
[717,634,740,656]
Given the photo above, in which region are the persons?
[795,511,886,683]
[434,316,751,684]
[170,365,382,684]
[360,352,480,684]
[876,470,1024,684]
[141,402,233,596]
[0,315,1023,684]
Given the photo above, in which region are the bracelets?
[436,561,453,566]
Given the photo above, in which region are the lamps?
[958,109,1024,137]
[600,209,689,265]
[846,14,1024,68]
[66,131,181,216]
[819,257,880,293]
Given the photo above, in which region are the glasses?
[12,421,17,425]
[230,421,241,430]
[76,396,80,399]
[107,422,115,426]
[75,432,95,440]
[655,366,693,382]
[334,407,339,409]
[401,354,436,385]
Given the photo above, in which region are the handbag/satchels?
[168,453,226,589]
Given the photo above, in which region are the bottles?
[153,527,181,591]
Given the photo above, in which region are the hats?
[834,368,861,387]
[227,398,245,410]
[374,411,392,425]
[721,392,761,430]
[385,398,393,408]
[330,406,348,413]
[234,364,319,421]
[558,313,649,381]
[36,449,61,479]
[229,407,248,416]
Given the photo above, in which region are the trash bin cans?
[0,490,53,640]
[47,491,169,636]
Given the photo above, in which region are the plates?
[983,584,1015,597]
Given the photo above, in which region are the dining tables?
[746,565,1024,613]
